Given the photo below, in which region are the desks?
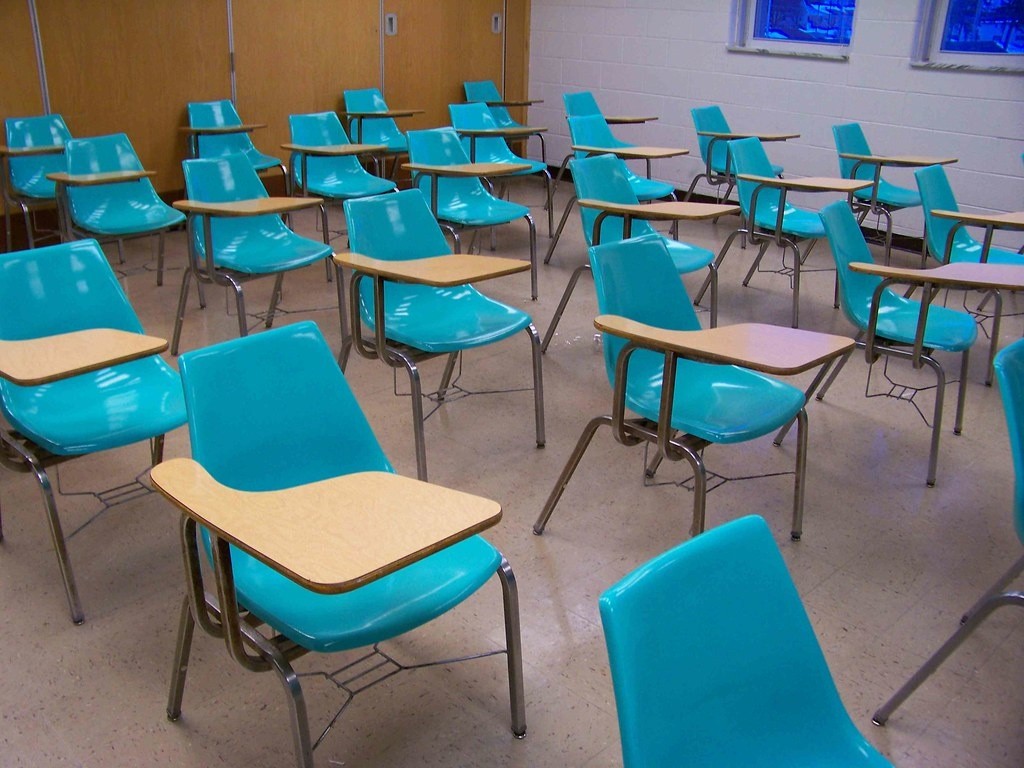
[849,261,1024,370]
[0,146,65,208]
[593,312,857,463]
[604,114,660,127]
[737,174,876,250]
[571,144,690,203]
[838,152,959,215]
[344,109,427,145]
[0,328,170,388]
[401,161,531,223]
[46,171,159,240]
[930,209,1024,265]
[178,121,268,160]
[695,131,801,185]
[577,199,742,241]
[151,458,504,674]
[333,248,533,365]
[283,143,389,195]
[173,197,327,288]
[457,128,549,164]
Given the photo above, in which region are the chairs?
[541,151,719,355]
[345,88,409,179]
[772,200,978,487]
[902,164,1024,300]
[57,130,208,310]
[170,151,347,357]
[564,113,681,243]
[405,126,539,302]
[187,98,295,232]
[696,136,840,328]
[287,110,400,281]
[166,319,529,768]
[448,102,555,250]
[336,189,548,484]
[564,90,653,206]
[797,121,923,281]
[533,234,809,540]
[0,112,126,265]
[463,80,546,162]
[670,105,784,249]
[594,512,897,768]
[0,236,189,626]
